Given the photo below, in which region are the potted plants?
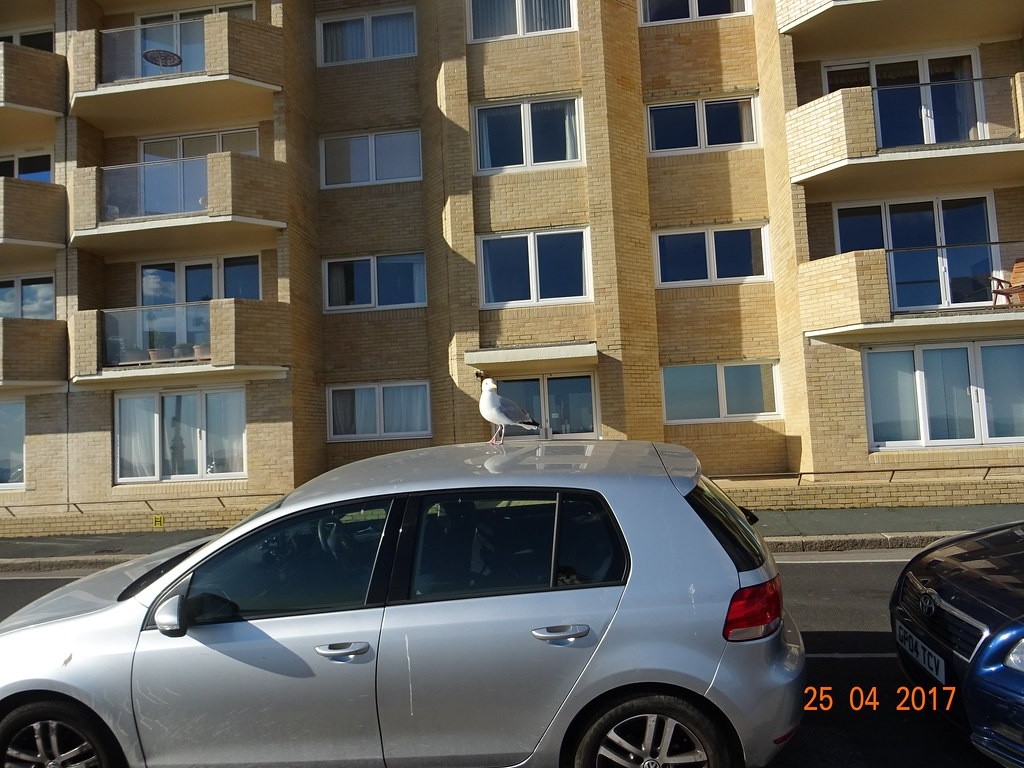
[146,332,174,365]
[171,343,194,363]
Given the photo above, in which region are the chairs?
[436,502,481,582]
[988,258,1024,310]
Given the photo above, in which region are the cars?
[886,517,1023,768]
[0,430,809,767]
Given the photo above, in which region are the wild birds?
[479,378,539,445]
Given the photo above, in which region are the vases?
[192,343,212,359]
[121,347,145,361]
